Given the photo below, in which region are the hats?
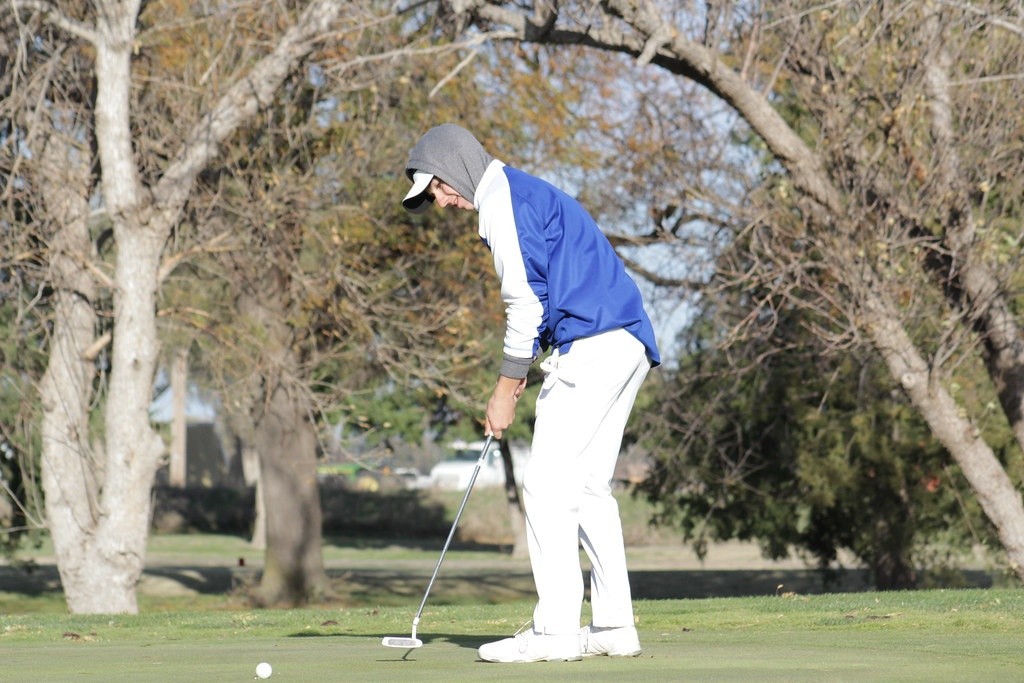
[400,169,434,210]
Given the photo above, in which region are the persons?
[400,123,662,663]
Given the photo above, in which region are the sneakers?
[476,621,583,666]
[571,623,643,657]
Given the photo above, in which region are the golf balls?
[255,662,272,680]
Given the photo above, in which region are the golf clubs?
[382,430,495,649]
